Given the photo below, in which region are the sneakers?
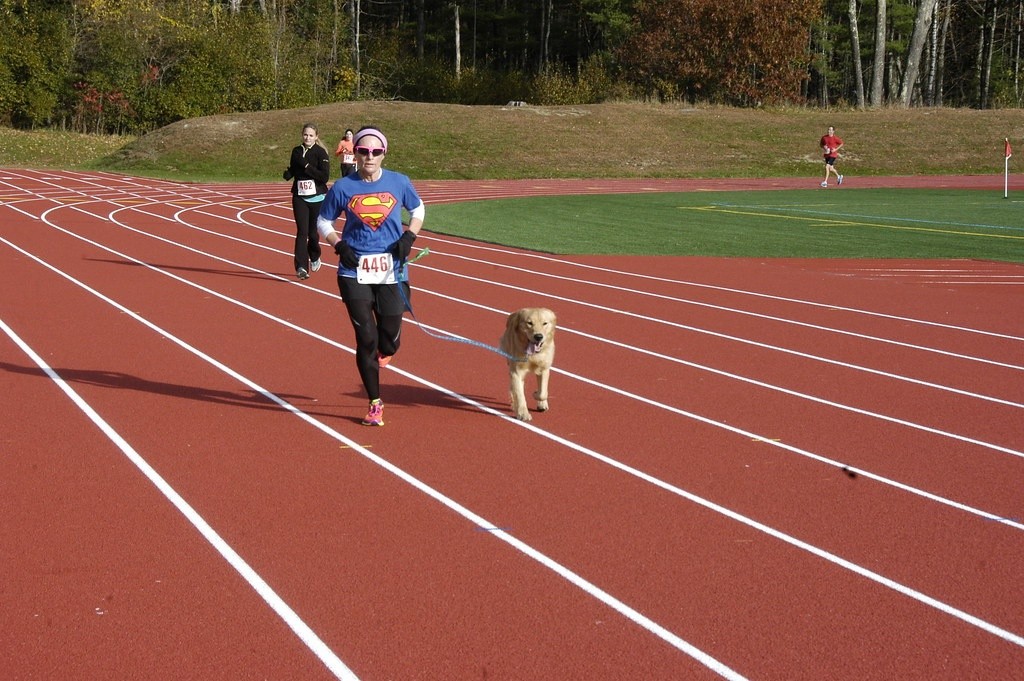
[311,257,321,272]
[362,401,384,426]
[821,181,828,188]
[837,175,843,185]
[297,268,310,280]
[377,352,393,368]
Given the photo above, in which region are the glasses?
[355,146,385,156]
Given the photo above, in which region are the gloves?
[390,230,417,262]
[297,156,308,167]
[283,171,293,180]
[335,240,359,269]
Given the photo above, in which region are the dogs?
[504,307,555,422]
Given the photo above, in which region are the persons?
[316,125,426,426]
[282,122,330,280]
[820,125,844,188]
